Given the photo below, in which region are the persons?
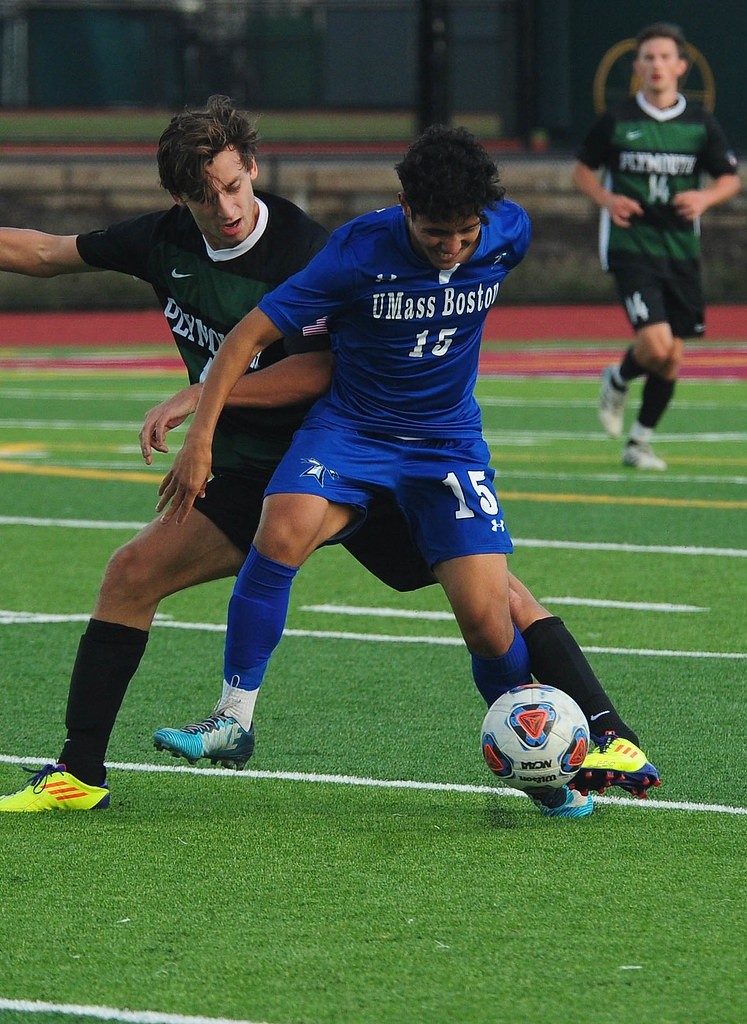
[0,94,661,812]
[574,22,741,473]
[154,124,594,823]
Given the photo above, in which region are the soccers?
[480,683,591,793]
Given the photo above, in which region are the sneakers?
[625,445,667,471]
[567,734,663,799]
[598,367,629,437]
[0,764,110,813]
[525,782,594,817]
[153,675,256,771]
